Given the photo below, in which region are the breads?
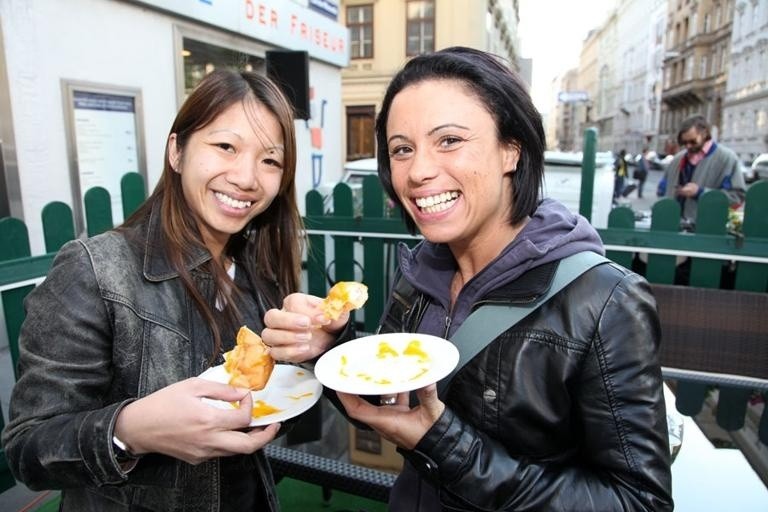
[319,279,369,325]
[220,326,272,397]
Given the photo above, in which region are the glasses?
[681,130,702,145]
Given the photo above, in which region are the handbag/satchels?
[633,170,646,180]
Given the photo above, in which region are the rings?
[381,396,398,404]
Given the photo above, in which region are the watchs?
[112,424,142,462]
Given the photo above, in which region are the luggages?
[623,183,638,197]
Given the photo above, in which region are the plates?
[313,332,459,396]
[197,361,323,429]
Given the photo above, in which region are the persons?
[1,72,326,512]
[615,112,768,230]
[262,44,674,510]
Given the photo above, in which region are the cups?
[666,408,685,465]
